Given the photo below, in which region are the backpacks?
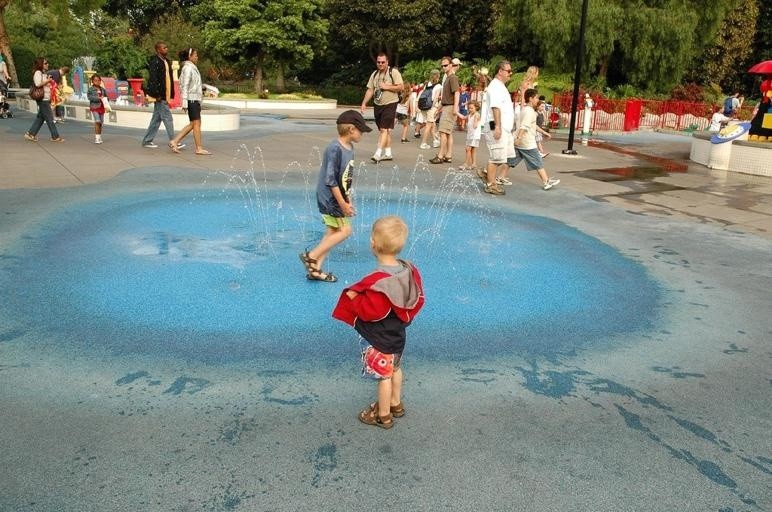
[418,82,442,110]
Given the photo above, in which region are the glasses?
[502,68,510,73]
[441,64,448,68]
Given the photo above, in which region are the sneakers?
[25,132,38,142]
[475,168,512,195]
[371,155,381,162]
[50,137,64,142]
[419,142,431,149]
[401,138,410,143]
[144,142,158,147]
[542,179,560,189]
[380,155,392,161]
[540,152,550,158]
[432,140,441,148]
[168,141,186,154]
[94,137,103,144]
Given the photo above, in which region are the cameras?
[375,87,383,100]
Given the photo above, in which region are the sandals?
[429,154,452,163]
[358,407,394,429]
[305,272,337,282]
[298,251,322,280]
[371,401,405,418]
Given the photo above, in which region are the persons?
[300,108,373,283]
[0,90,7,114]
[24,57,70,143]
[141,41,186,150]
[166,48,212,155]
[0,54,12,84]
[87,73,109,144]
[722,88,745,126]
[332,214,425,429]
[749,74,772,138]
[709,103,734,132]
[360,52,561,195]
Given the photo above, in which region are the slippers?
[195,150,212,155]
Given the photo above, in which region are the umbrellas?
[748,60,772,74]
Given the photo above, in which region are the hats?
[336,110,373,132]
[451,58,463,65]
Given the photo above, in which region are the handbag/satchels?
[28,83,44,100]
